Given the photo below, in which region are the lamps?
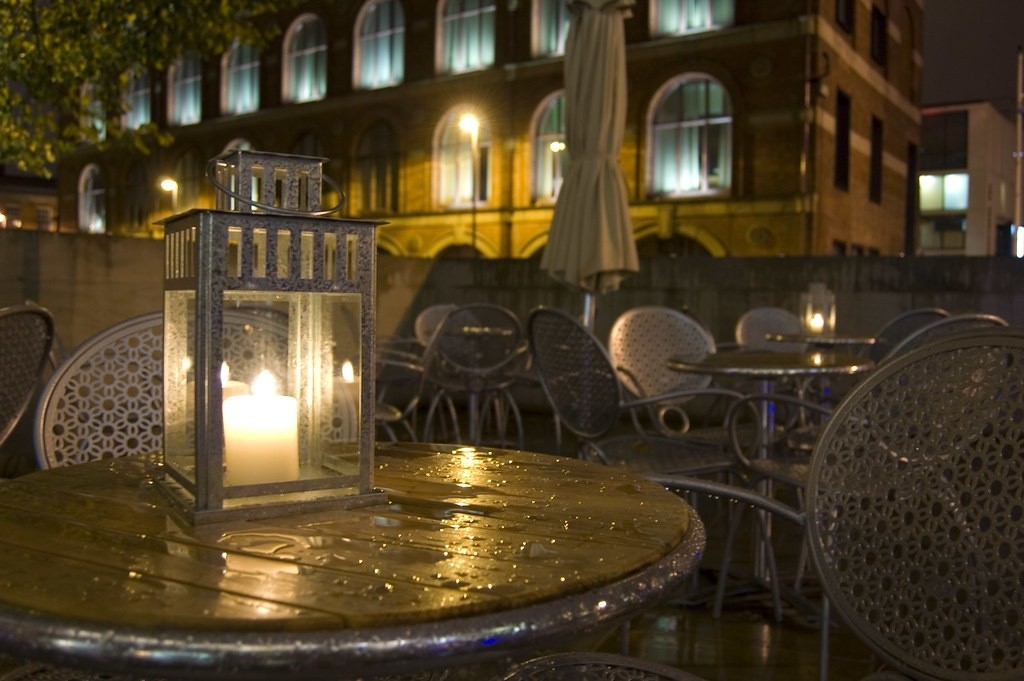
[800,283,837,336]
[151,149,389,525]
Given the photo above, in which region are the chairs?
[0,304,1024,681]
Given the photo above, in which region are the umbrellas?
[543,0,636,461]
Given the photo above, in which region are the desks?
[766,331,874,358]
[665,351,876,621]
[0,440,703,681]
